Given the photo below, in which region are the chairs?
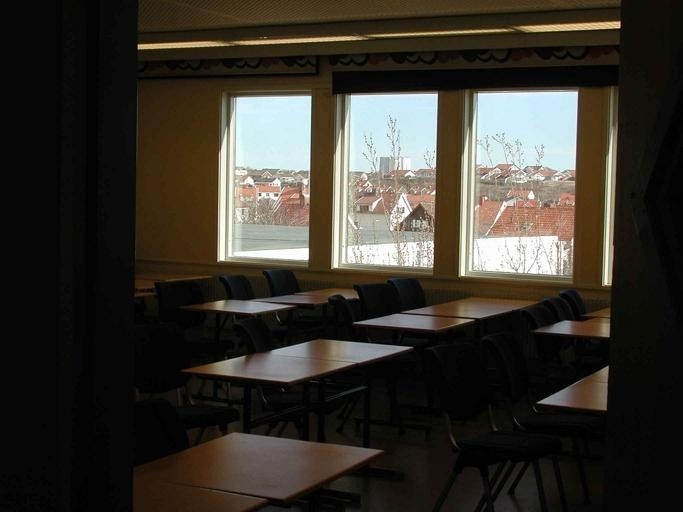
[432,288,589,509]
[154,268,427,355]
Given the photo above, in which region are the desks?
[353,297,538,440]
[531,305,608,338]
[131,432,385,510]
[136,274,212,299]
[180,338,415,449]
[536,365,608,411]
